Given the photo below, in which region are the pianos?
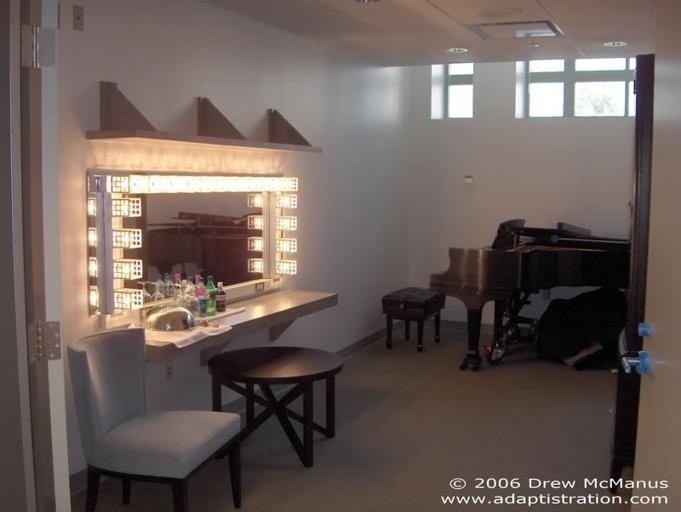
[430,220,629,371]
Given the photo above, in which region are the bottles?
[182,275,226,317]
[155,272,193,296]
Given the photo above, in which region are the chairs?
[66,326,243,512]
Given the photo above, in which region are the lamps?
[82,166,300,318]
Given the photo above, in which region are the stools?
[381,286,446,353]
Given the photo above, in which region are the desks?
[207,346,344,467]
